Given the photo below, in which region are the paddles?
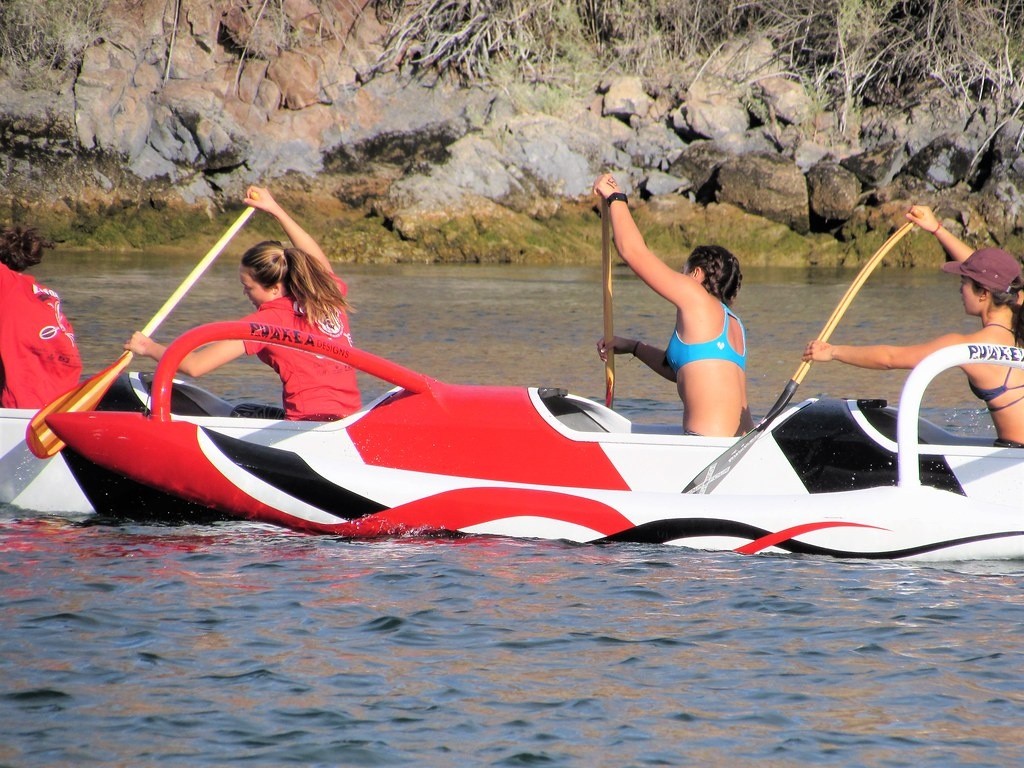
[25,191,261,458]
[681,220,916,495]
[597,189,615,409]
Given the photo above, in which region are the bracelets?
[606,192,628,203]
[932,221,942,234]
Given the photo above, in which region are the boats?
[1,322,1024,563]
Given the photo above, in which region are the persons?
[591,173,756,438]
[0,220,82,408]
[123,185,364,421]
[801,204,1024,451]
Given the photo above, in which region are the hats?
[939,247,1023,295]
